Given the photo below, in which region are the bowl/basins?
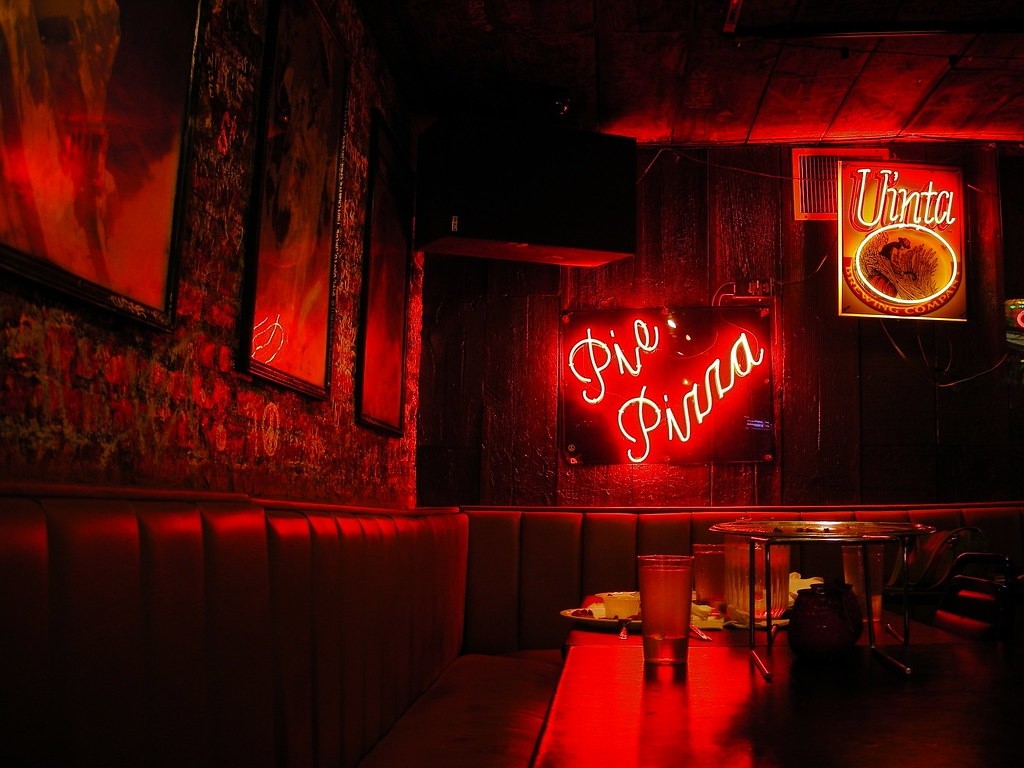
[595,591,640,620]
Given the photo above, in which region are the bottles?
[788,583,864,658]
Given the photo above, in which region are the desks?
[523,608,1024,768]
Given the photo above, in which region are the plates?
[560,608,642,630]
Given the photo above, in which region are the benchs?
[0,479,1024,768]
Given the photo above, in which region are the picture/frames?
[237,0,356,403]
[0,0,211,331]
[354,109,416,437]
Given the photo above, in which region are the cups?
[693,543,727,614]
[638,555,695,665]
[724,532,789,620]
[842,545,883,622]
